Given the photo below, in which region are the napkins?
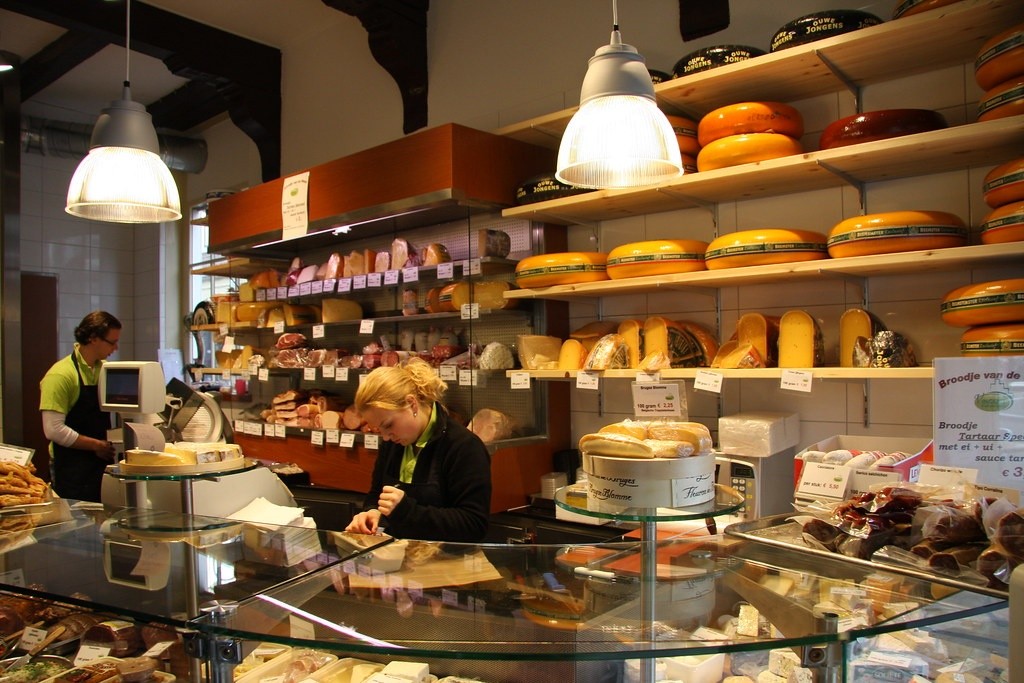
[226,496,322,567]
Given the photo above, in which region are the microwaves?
[714,447,797,520]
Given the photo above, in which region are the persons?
[344,357,492,544]
[38,310,123,502]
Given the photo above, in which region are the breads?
[1,229,1024,683]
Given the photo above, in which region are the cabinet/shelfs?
[232,256,551,515]
[190,216,290,375]
[496,0,1024,380]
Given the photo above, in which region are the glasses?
[100,337,119,346]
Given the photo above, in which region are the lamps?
[555,0,684,189]
[64,0,182,224]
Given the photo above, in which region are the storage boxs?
[305,658,438,683]
[555,488,613,525]
[582,450,718,508]
[234,649,339,683]
[794,435,934,499]
